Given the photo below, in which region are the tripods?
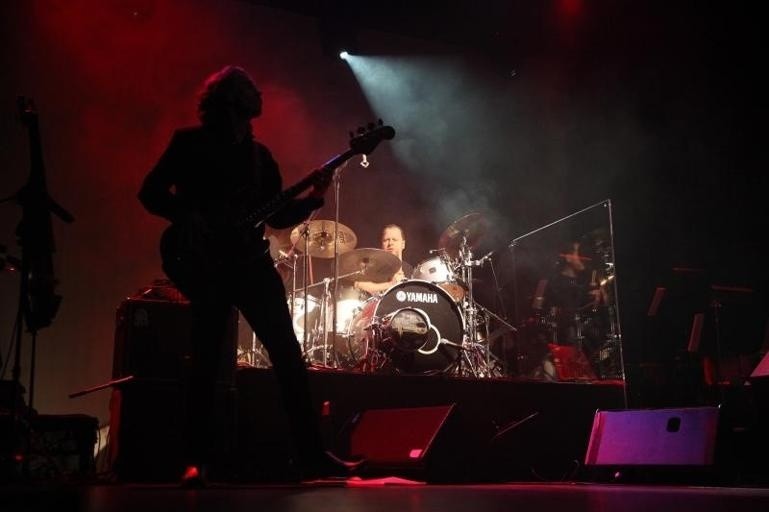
[452,267,496,379]
[237,269,367,366]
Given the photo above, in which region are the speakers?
[108,297,240,481]
[335,401,458,471]
[585,405,719,468]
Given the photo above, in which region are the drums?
[320,299,371,351]
[347,279,463,376]
[287,289,322,356]
[411,256,465,303]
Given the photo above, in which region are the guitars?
[159,120,395,302]
[331,248,402,282]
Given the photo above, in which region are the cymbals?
[438,213,487,254]
[290,220,357,259]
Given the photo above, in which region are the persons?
[132,62,370,489]
[349,218,416,300]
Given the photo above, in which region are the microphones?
[440,337,466,353]
